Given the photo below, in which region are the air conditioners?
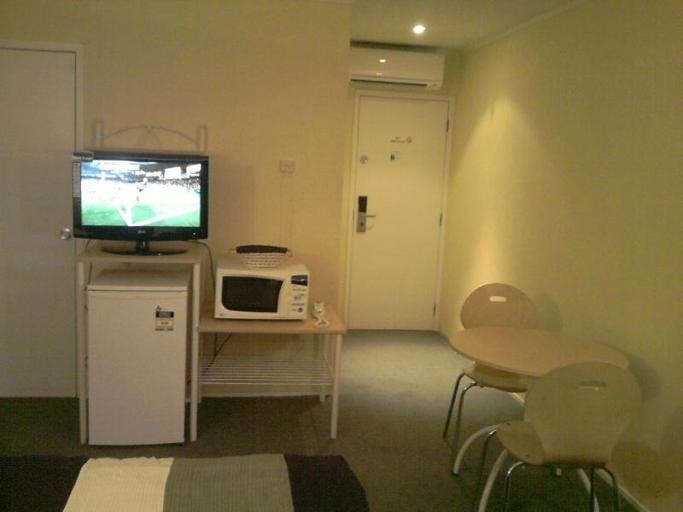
[349,39,446,92]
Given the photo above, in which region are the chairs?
[469,359,631,511]
[440,282,539,453]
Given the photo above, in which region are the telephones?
[312,301,325,318]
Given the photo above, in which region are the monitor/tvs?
[72,150,209,256]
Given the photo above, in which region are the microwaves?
[214,255,309,320]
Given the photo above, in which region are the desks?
[447,326,631,511]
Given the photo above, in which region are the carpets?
[0,453,370,512]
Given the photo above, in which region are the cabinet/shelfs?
[74,240,201,442]
[198,300,345,441]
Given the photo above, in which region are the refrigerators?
[87,268,191,445]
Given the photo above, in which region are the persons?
[118,172,200,205]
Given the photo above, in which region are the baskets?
[227,242,292,270]
